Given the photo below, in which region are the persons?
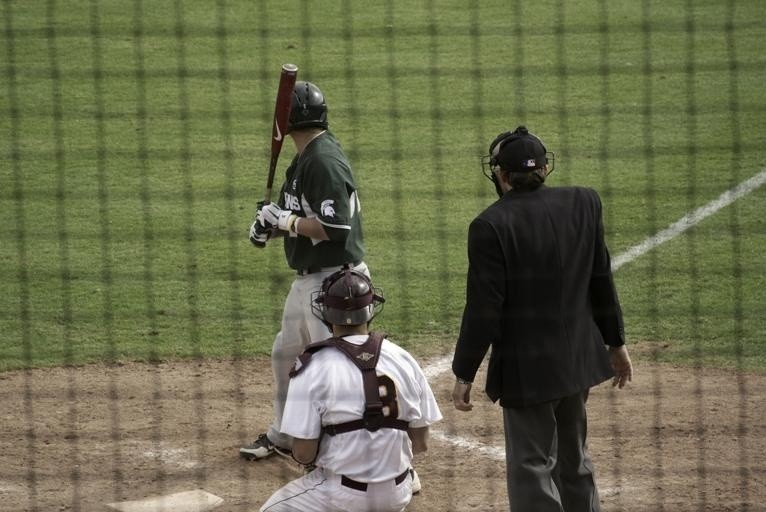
[258,267,444,512]
[449,125,632,512]
[238,81,372,462]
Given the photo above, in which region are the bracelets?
[455,377,471,385]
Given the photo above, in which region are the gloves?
[249,221,268,249]
[256,201,293,230]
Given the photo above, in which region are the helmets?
[284,81,327,134]
[480,126,555,197]
[310,267,385,326]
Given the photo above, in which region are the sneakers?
[411,469,421,493]
[239,434,287,461]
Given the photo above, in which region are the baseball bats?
[265,64,299,205]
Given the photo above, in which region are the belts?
[308,261,361,275]
[342,468,409,491]
